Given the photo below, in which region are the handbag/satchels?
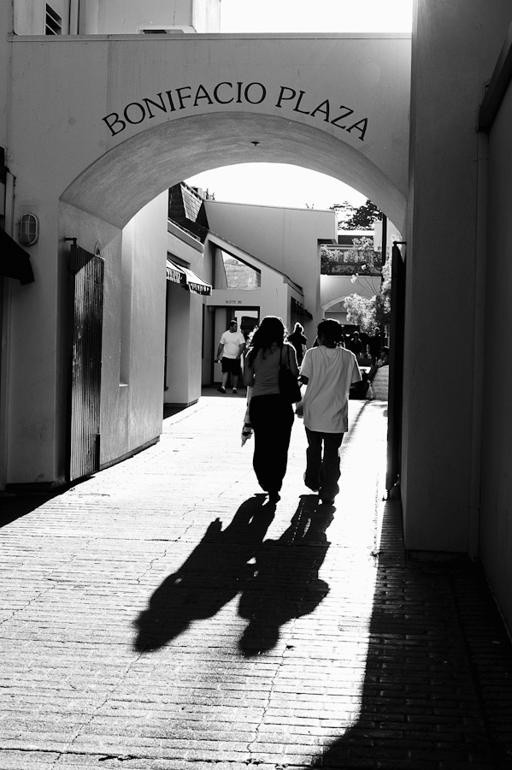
[279,367,302,403]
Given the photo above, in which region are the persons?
[298,319,363,508]
[242,314,299,502]
[216,319,245,393]
[286,322,307,370]
[337,324,385,368]
